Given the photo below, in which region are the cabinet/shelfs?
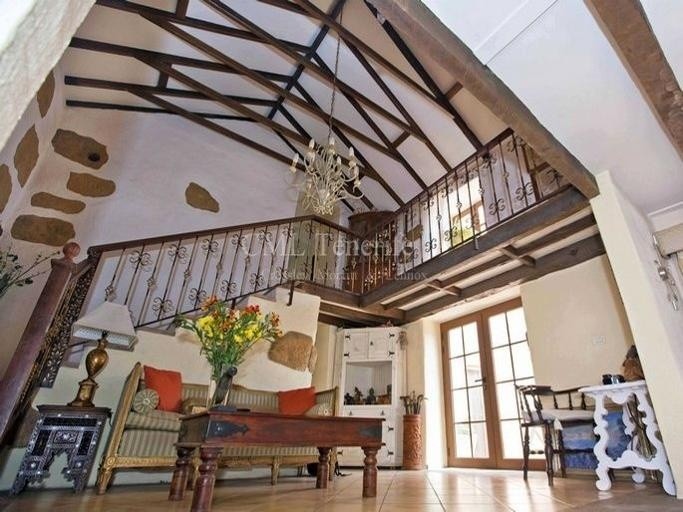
[331,327,408,469]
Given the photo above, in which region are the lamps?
[283,0,366,216]
[67,301,139,409]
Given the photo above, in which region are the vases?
[206,363,240,411]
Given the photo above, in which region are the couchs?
[95,361,340,495]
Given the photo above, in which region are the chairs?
[514,385,616,486]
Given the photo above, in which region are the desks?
[577,379,677,496]
[6,404,112,497]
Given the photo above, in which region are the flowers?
[172,293,283,387]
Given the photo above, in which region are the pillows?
[276,386,334,417]
[132,365,182,414]
